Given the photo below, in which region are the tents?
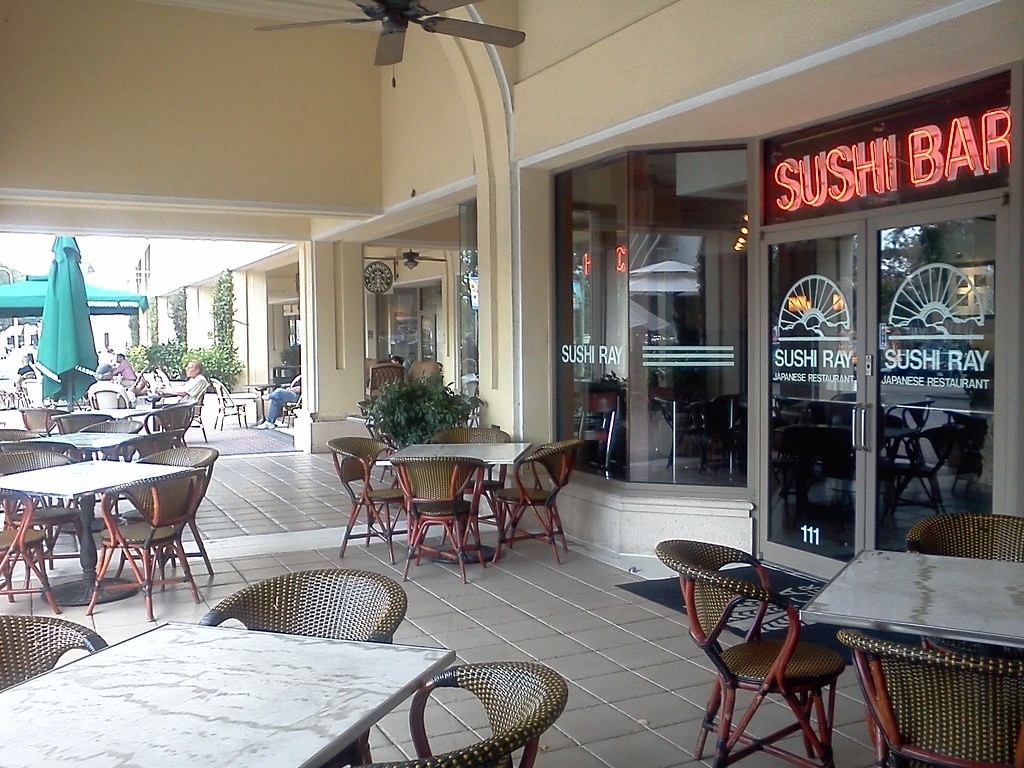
[0,281,149,349]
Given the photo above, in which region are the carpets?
[614,564,922,666]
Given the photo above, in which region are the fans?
[362,248,447,264]
[253,0,526,89]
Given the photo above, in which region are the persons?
[18,356,35,379]
[391,352,416,368]
[155,360,207,415]
[257,374,302,429]
[87,353,149,409]
[461,333,478,363]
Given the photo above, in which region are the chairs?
[656,540,854,768]
[199,569,408,768]
[574,391,620,477]
[836,629,1024,768]
[651,387,987,525]
[408,360,441,385]
[429,428,511,545]
[282,394,301,428]
[906,513,1024,659]
[326,436,422,566]
[0,369,211,622]
[366,363,404,402]
[353,662,568,768]
[390,457,487,584]
[492,438,585,564]
[210,379,249,431]
[0,615,108,692]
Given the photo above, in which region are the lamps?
[955,250,964,259]
[788,295,811,312]
[833,294,844,311]
[403,261,419,269]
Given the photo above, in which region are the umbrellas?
[33,235,98,412]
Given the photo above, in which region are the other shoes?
[261,394,270,401]
[257,421,275,429]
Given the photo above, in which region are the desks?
[376,443,533,563]
[0,459,196,606]
[136,392,177,432]
[799,548,1024,752]
[20,432,143,534]
[242,383,282,430]
[71,408,159,501]
[0,622,456,768]
[774,422,920,511]
